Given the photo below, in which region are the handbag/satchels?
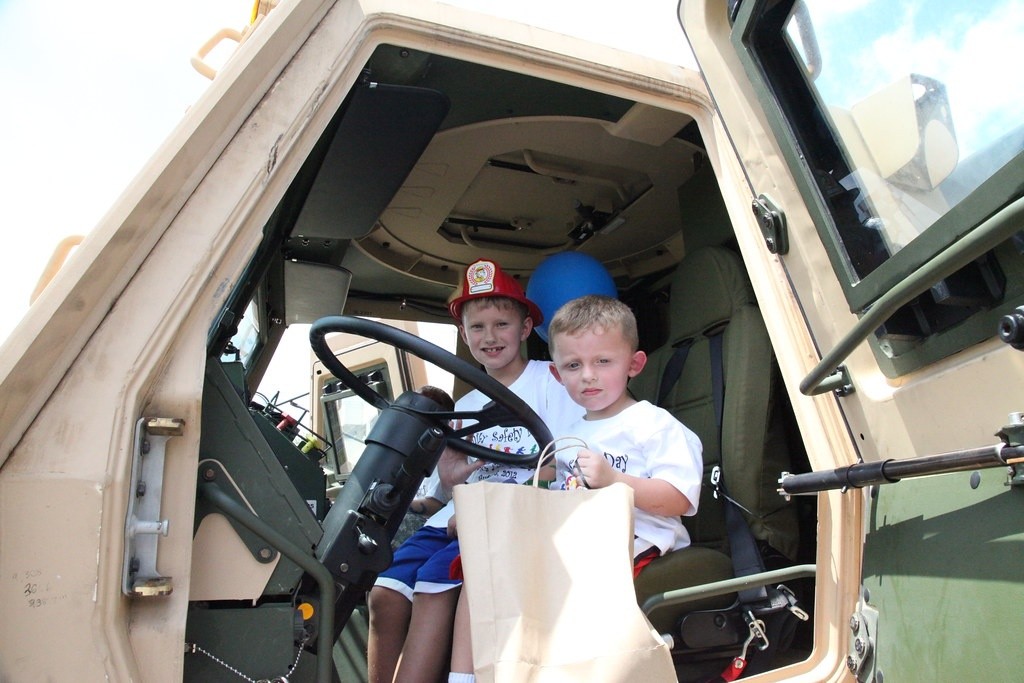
[451,436,678,683]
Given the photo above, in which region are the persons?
[366,259,586,683]
[448,297,704,683]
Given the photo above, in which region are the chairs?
[625,243,803,636]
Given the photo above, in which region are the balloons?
[526,251,622,345]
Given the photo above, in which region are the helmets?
[449,257,544,327]
